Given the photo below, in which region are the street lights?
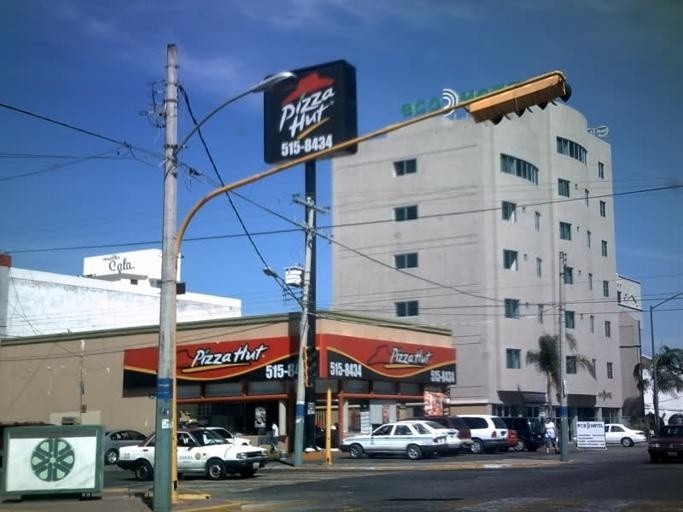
[264,265,306,465]
[155,71,296,511]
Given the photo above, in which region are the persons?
[541,417,559,454]
[270,421,279,453]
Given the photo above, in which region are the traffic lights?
[462,69,571,125]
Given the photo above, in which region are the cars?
[397,420,463,451]
[102,428,147,464]
[570,423,647,448]
[191,426,251,446]
[502,429,518,450]
[118,428,269,481]
[340,422,447,460]
[647,424,682,463]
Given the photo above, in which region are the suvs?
[401,416,473,451]
[501,416,545,452]
[451,415,507,454]
[0,420,56,470]
